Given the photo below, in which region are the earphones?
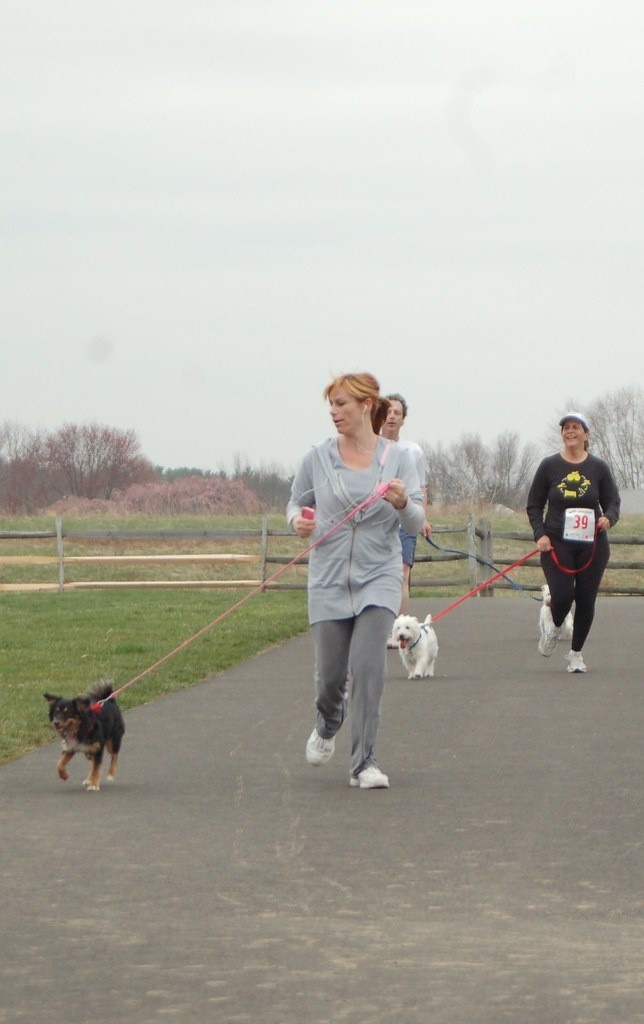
[363,404,367,414]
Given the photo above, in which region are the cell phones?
[301,507,315,520]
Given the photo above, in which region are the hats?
[559,411,591,432]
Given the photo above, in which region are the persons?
[526,413,621,673]
[381,393,431,648]
[286,373,425,789]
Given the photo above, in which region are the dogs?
[43,678,125,791]
[391,613,438,679]
[539,584,574,640]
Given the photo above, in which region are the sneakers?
[304,727,337,767]
[565,651,588,673]
[537,620,566,657]
[349,764,390,789]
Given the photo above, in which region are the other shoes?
[388,639,399,649]
